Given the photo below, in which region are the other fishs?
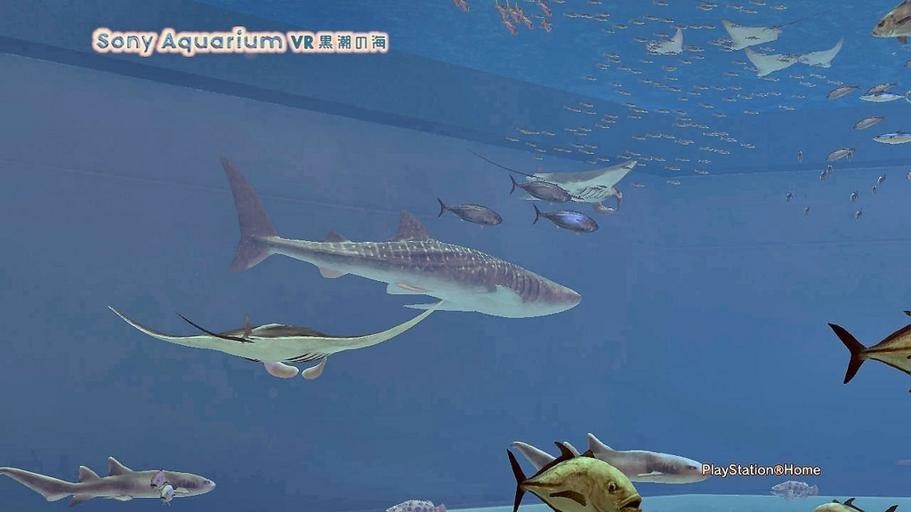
[437,0,911,232]
